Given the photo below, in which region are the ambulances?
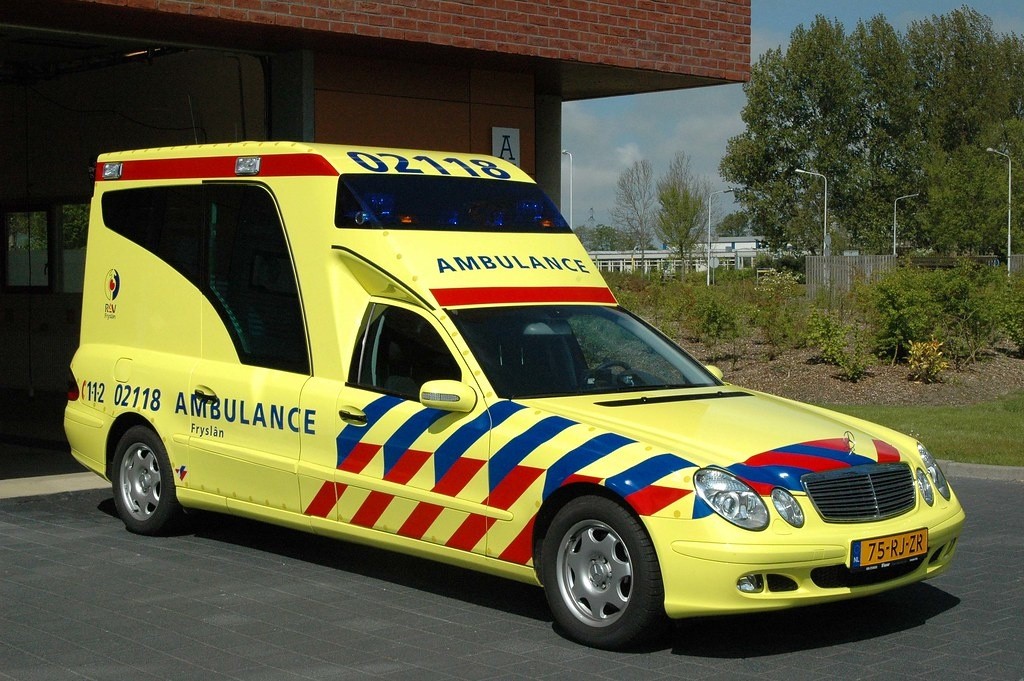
[60,140,965,653]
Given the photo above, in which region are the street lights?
[892,193,920,254]
[563,150,573,230]
[795,169,826,256]
[987,147,1012,279]
[707,189,733,286]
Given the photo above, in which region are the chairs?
[387,329,444,390]
[489,311,536,396]
